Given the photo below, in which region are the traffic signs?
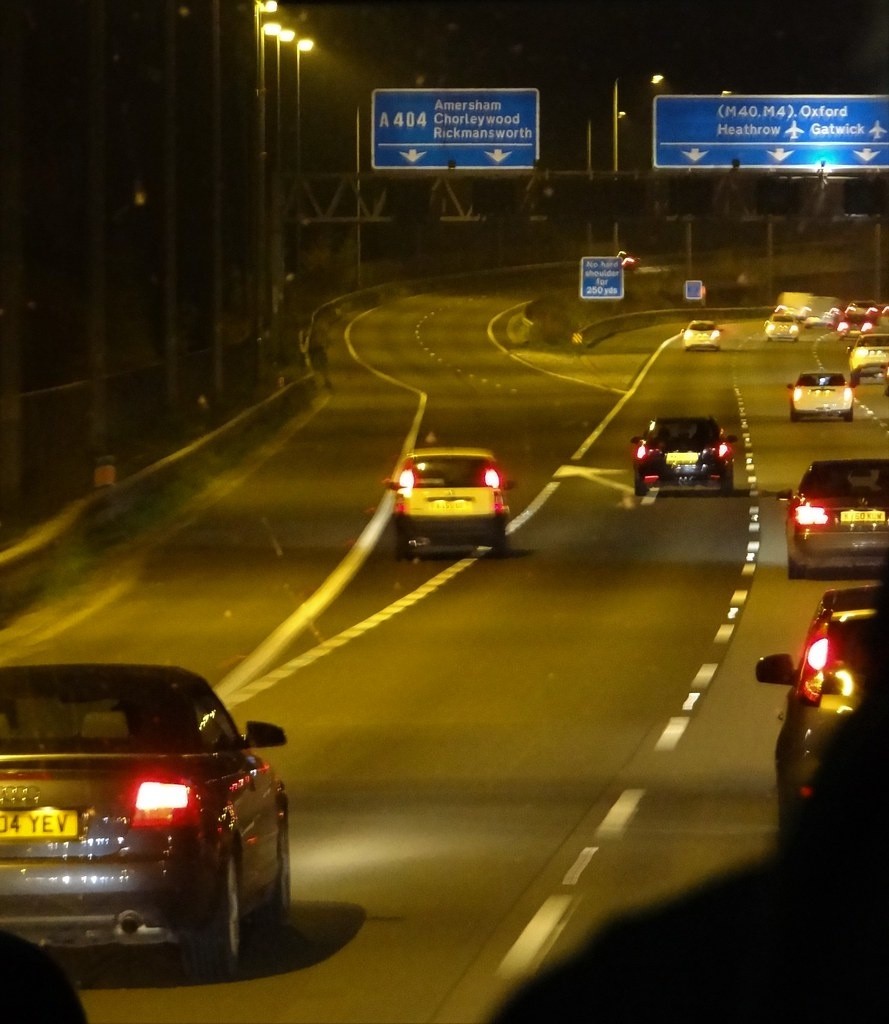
[651,91,889,172]
[370,85,542,172]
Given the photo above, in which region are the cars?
[786,370,857,421]
[754,582,889,839]
[775,455,889,584]
[847,332,889,388]
[0,662,296,979]
[629,415,740,496]
[380,446,519,562]
[799,297,889,341]
[763,311,800,342]
[680,319,724,352]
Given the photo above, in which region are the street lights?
[584,110,628,258]
[253,0,317,379]
[609,74,664,253]
